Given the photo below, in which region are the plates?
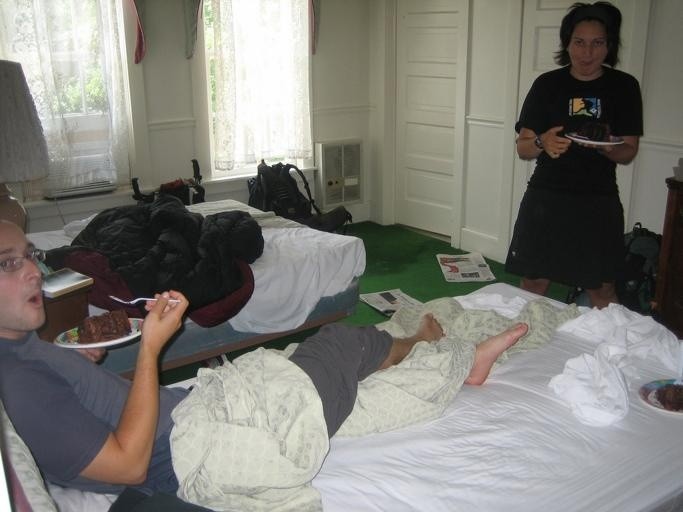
[52,316,144,348]
[637,378,683,417]
[565,133,625,147]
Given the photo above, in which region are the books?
[42,267,94,299]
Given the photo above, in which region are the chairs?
[247,159,353,235]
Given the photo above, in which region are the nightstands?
[35,285,91,344]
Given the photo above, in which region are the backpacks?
[257,163,319,217]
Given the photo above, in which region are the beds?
[1,285,683,512]
[22,199,366,382]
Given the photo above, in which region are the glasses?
[0,248,47,271]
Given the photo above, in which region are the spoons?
[109,294,181,306]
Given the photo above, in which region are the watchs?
[535,135,544,152]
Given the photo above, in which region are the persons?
[505,1,645,310]
[1,219,528,496]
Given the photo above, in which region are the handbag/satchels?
[622,228,662,312]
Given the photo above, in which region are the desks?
[652,175,683,341]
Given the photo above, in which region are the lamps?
[0,59,50,233]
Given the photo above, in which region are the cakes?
[575,118,612,141]
[656,383,683,412]
[75,307,132,344]
